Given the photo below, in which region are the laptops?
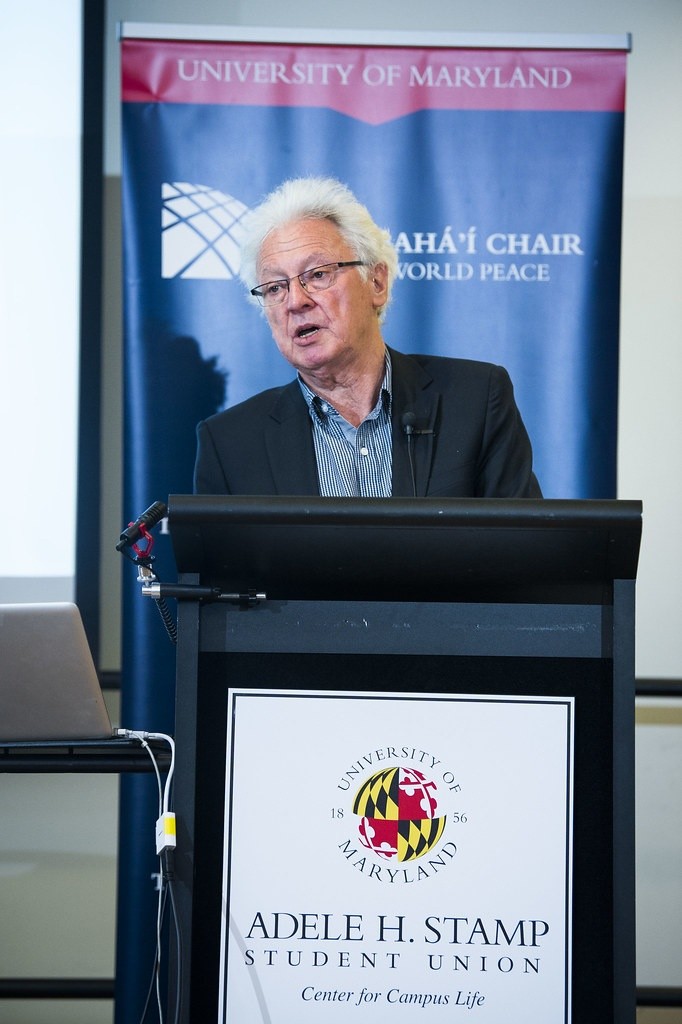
[0,602,124,741]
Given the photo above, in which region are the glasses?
[250,259,367,308]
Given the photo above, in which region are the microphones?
[117,501,167,552]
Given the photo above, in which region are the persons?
[193,177,543,499]
